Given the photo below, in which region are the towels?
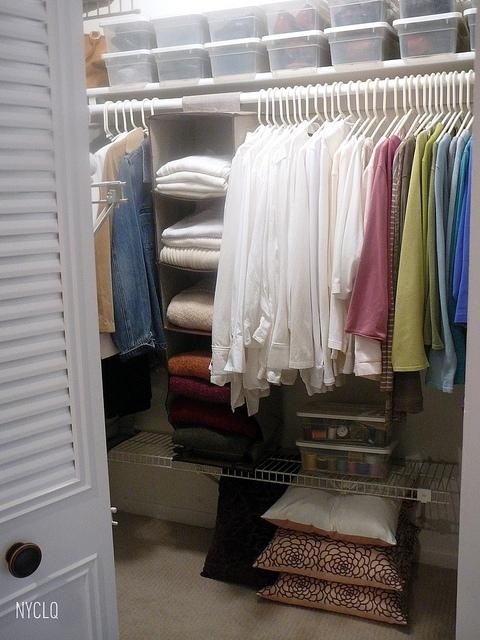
[155,153,233,199]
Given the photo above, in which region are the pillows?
[199,454,421,626]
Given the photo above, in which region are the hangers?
[90,98,158,169]
[234,69,475,171]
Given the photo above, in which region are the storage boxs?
[295,400,398,479]
